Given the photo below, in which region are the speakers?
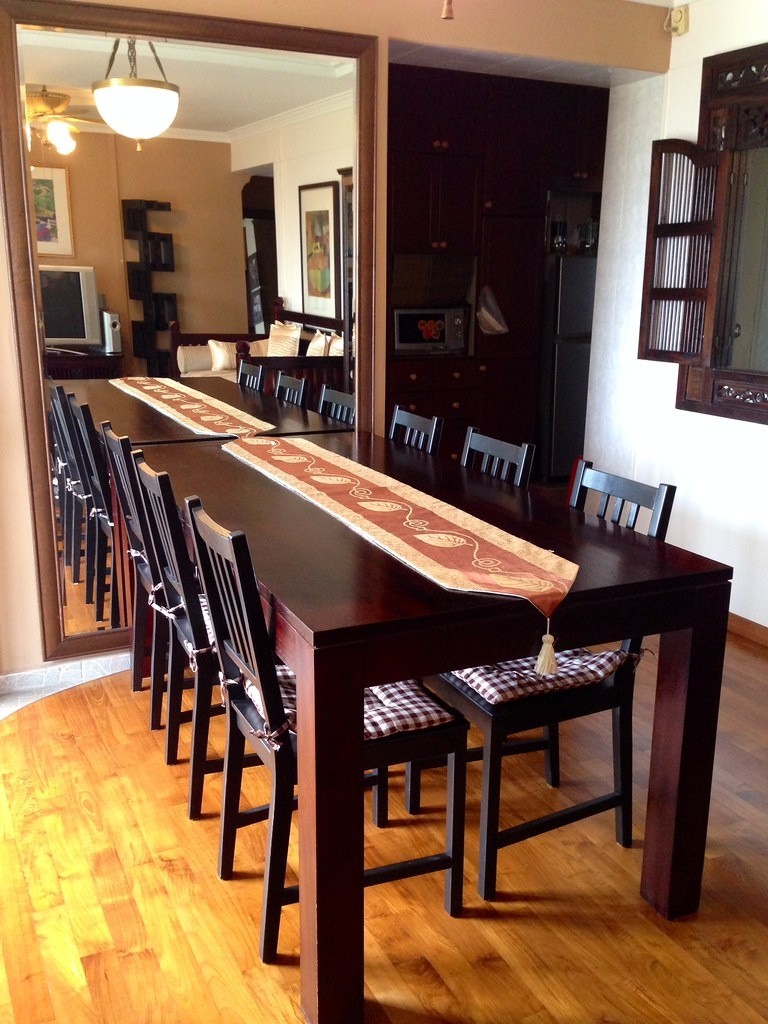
[104,311,122,353]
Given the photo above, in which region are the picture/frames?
[29,164,76,260]
[298,181,339,334]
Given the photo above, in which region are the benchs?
[169,297,358,408]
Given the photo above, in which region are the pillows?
[176,320,345,371]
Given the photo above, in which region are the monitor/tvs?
[37,265,102,355]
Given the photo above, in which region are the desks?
[117,431,733,1024]
[46,377,357,445]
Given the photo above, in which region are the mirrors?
[0,2,375,666]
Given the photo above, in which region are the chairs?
[99,404,678,962]
[45,358,358,622]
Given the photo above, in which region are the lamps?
[91,33,181,153]
[20,90,75,155]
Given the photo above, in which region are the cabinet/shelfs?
[386,61,611,487]
